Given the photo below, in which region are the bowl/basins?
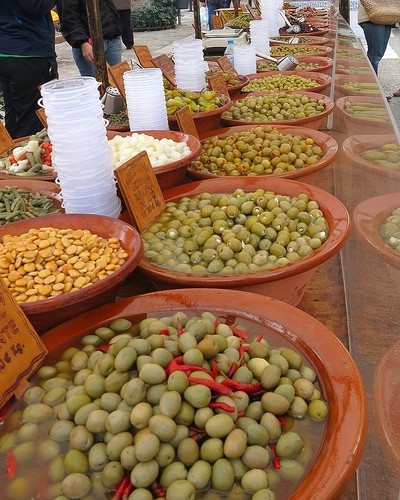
[269,44,332,56]
[0,130,121,180]
[0,211,143,333]
[187,124,338,182]
[242,71,332,93]
[247,0,331,46]
[0,178,66,223]
[118,175,350,308]
[116,129,202,201]
[233,46,256,76]
[256,56,334,73]
[1,286,368,500]
[336,95,393,135]
[227,74,250,94]
[167,89,232,134]
[350,192,400,269]
[332,47,381,95]
[41,76,123,218]
[219,90,335,130]
[372,339,400,483]
[171,37,207,92]
[122,67,170,131]
[106,123,130,130]
[338,132,400,193]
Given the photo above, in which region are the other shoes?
[385,95,392,101]
[392,88,400,96]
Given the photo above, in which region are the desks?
[120,14,399,498]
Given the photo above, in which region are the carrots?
[10,139,53,166]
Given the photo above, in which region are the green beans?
[0,186,62,226]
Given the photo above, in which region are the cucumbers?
[3,128,47,176]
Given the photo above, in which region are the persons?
[0,0,60,139]
[207,0,231,31]
[357,0,392,101]
[199,0,210,31]
[56,0,123,79]
[112,0,135,49]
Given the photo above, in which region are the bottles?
[223,40,237,67]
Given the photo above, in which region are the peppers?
[0,450,51,500]
[94,317,285,468]
[165,87,227,115]
[108,472,168,500]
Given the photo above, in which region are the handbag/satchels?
[360,0,400,24]
[200,6,208,28]
